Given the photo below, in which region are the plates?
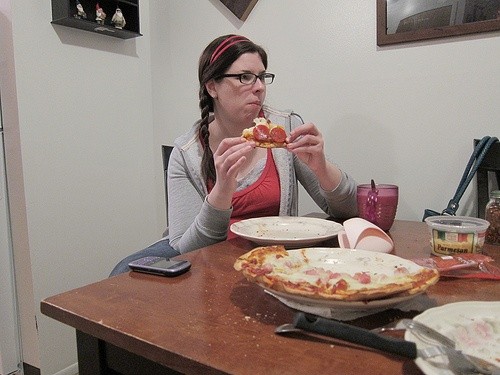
[256,247,422,312]
[404,301,500,375]
[230,216,343,249]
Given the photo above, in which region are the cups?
[357,184,398,233]
[425,216,490,258]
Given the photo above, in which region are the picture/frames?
[376,0,500,46]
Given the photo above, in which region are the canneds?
[485,190,500,246]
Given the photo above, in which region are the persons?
[108,32,358,278]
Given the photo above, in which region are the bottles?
[485,190,500,246]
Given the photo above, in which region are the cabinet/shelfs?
[50,0,144,39]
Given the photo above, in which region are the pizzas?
[234,245,440,301]
[240,117,287,148]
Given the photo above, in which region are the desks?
[40,211,500,375]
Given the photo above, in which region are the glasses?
[220,73,275,85]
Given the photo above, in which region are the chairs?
[475,138,500,218]
